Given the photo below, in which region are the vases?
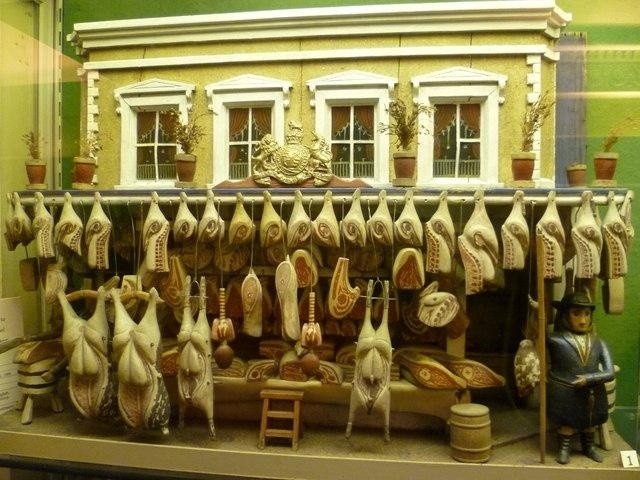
[566,164,588,189]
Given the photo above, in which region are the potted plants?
[594,117,636,186]
[376,93,437,188]
[70,130,100,187]
[160,101,214,190]
[20,130,49,191]
[510,83,558,187]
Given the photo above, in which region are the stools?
[258,388,306,451]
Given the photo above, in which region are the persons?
[546,290,616,464]
